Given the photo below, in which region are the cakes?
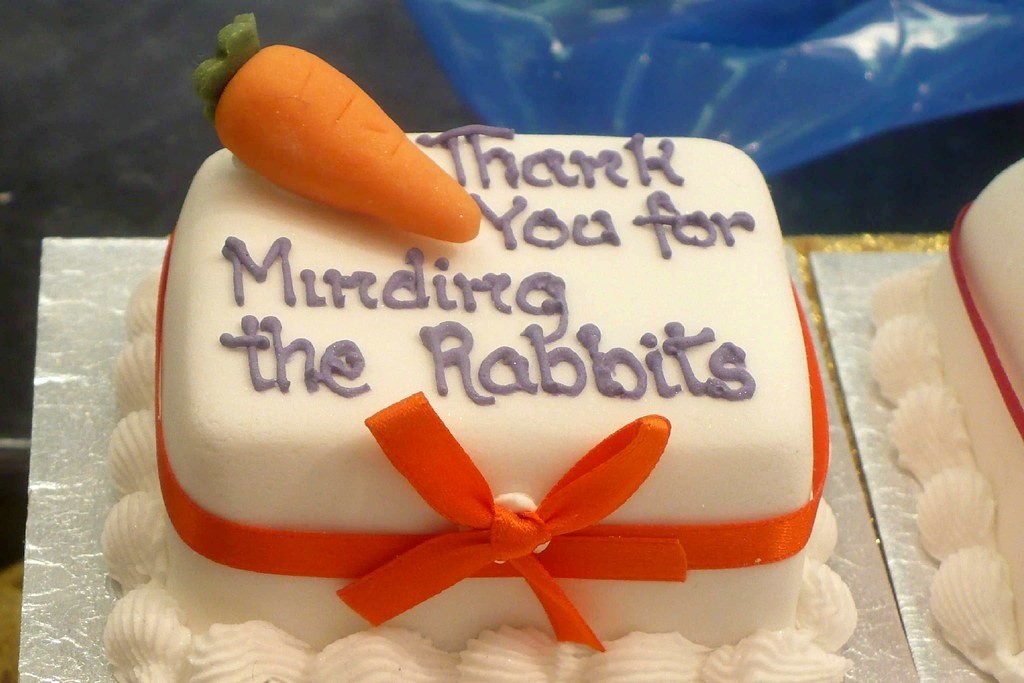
[98,14,863,682]
[867,152,1024,683]
